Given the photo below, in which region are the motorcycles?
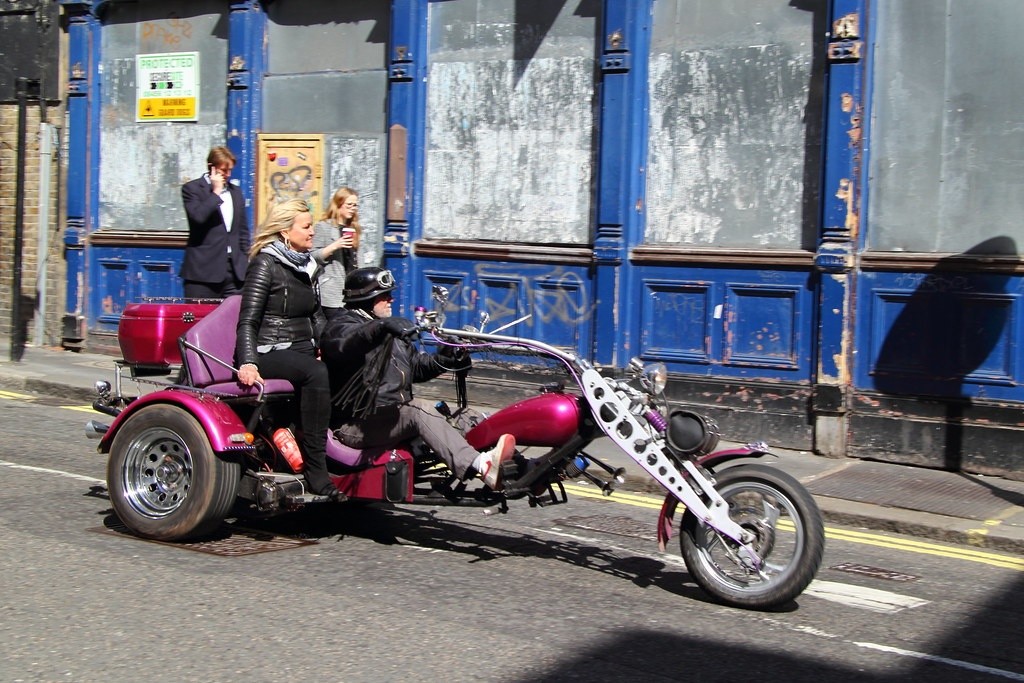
[85,300,826,612]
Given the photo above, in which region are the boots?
[301,386,336,496]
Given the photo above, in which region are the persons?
[234,186,516,495]
[180,146,250,304]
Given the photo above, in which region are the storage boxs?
[119,302,218,363]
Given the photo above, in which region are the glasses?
[343,269,396,297]
[342,202,360,210]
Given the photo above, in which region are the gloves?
[452,344,471,371]
[381,317,421,346]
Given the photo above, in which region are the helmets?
[342,267,399,302]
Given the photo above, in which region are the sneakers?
[476,432,515,491]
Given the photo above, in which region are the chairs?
[178,295,293,401]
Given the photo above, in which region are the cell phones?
[209,165,215,175]
